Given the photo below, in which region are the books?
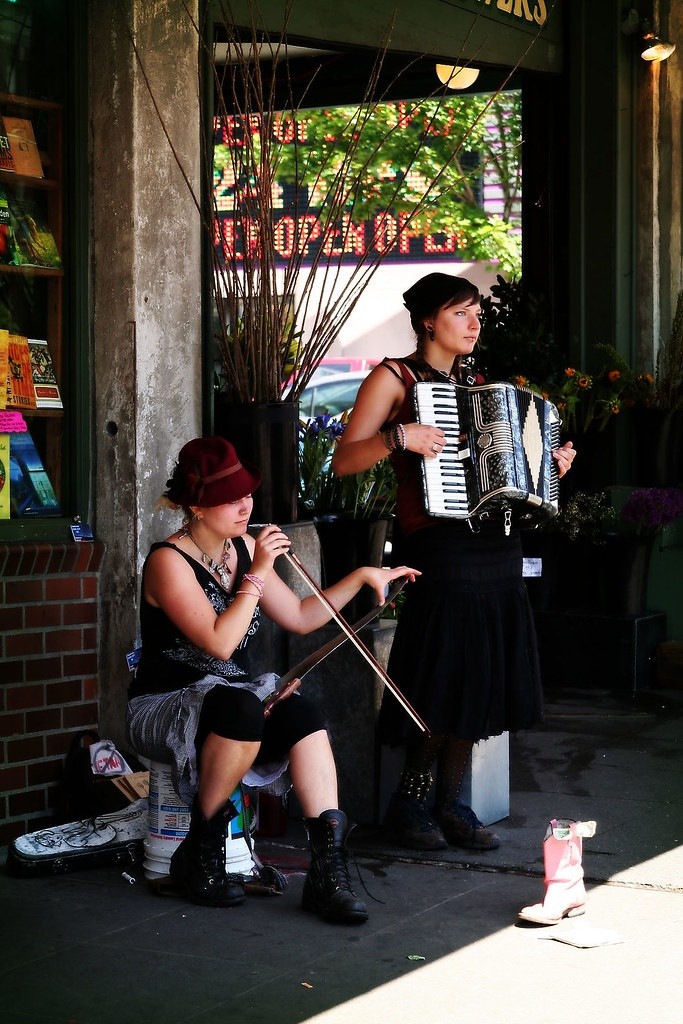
[0,115,64,521]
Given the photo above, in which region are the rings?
[433,444,438,450]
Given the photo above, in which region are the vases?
[214,391,303,525]
[313,508,395,624]
[519,429,655,617]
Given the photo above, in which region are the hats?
[167,438,262,508]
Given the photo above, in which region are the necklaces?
[425,360,458,383]
[178,525,232,590]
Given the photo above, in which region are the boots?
[431,736,500,849]
[378,722,449,849]
[518,818,588,925]
[169,798,245,905]
[301,808,383,920]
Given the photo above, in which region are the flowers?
[504,342,683,547]
[295,408,398,520]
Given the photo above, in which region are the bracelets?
[236,573,265,598]
[385,423,407,450]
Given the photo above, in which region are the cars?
[282,357,392,494]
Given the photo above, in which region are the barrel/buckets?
[142,761,254,880]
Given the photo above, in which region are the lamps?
[638,17,676,62]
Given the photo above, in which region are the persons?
[124,435,423,923]
[333,272,578,853]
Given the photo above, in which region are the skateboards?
[9,802,150,874]
